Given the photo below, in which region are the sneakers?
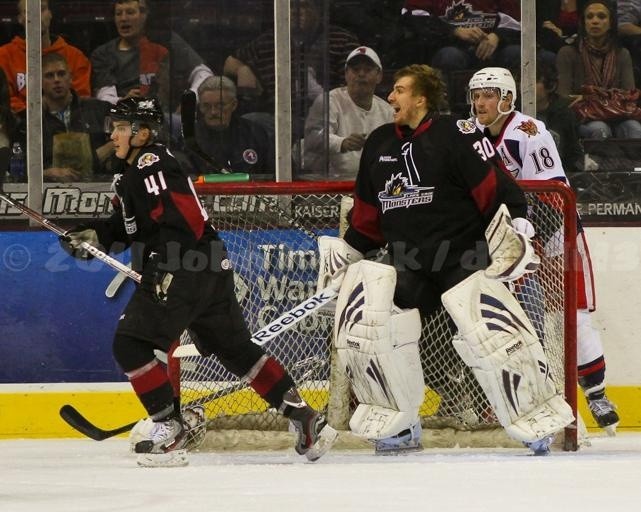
[295,410,325,454]
[376,423,421,450]
[525,436,551,452]
[585,388,619,427]
[137,419,185,452]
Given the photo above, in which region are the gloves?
[141,235,185,305]
[59,220,125,259]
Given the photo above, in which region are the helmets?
[467,67,518,130]
[106,97,164,148]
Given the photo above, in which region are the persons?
[60,96,319,459]
[465,66,622,433]
[319,62,575,452]
[1,0,640,181]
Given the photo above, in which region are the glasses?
[199,100,233,112]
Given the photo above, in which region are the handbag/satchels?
[569,86,640,121]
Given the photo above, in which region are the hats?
[344,46,383,71]
